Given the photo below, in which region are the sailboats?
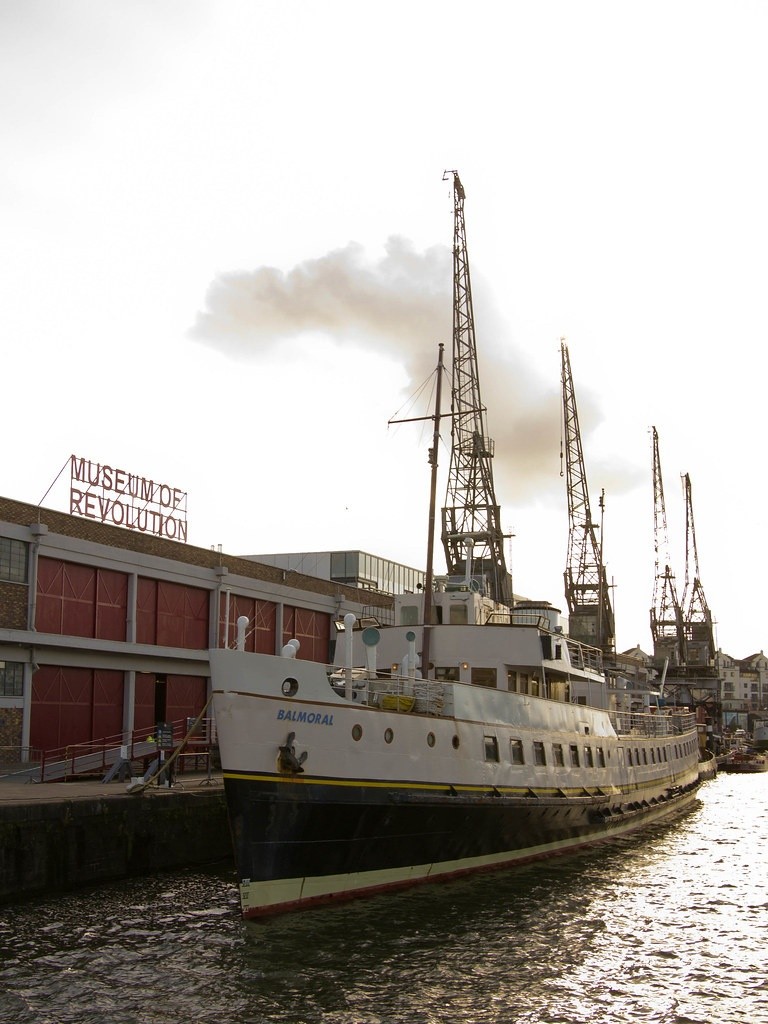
[207,341,704,920]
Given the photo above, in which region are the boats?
[697,715,768,782]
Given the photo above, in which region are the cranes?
[440,170,515,607]
[646,426,689,679]
[679,472,720,677]
[555,335,618,663]
